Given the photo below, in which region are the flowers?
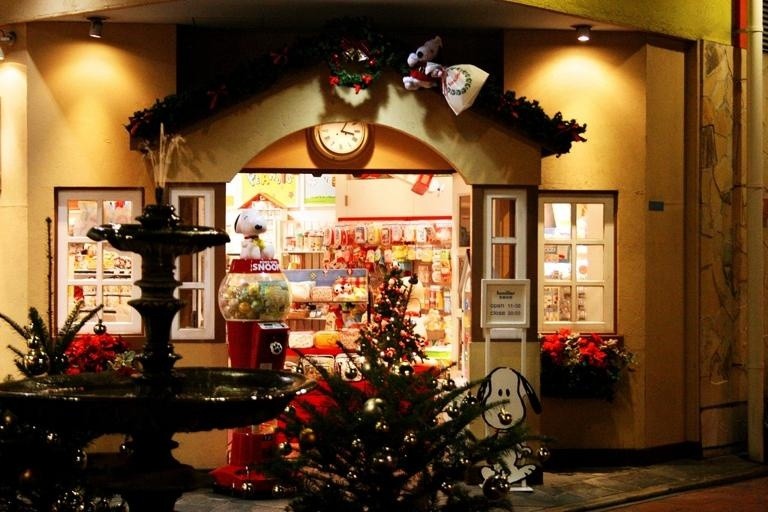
[542,330,640,383]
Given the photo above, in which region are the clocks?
[314,121,374,167]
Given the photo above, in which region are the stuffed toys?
[401,37,445,95]
[232,210,277,261]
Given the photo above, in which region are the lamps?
[576,25,591,42]
[87,14,106,39]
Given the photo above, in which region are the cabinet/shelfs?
[279,268,370,355]
